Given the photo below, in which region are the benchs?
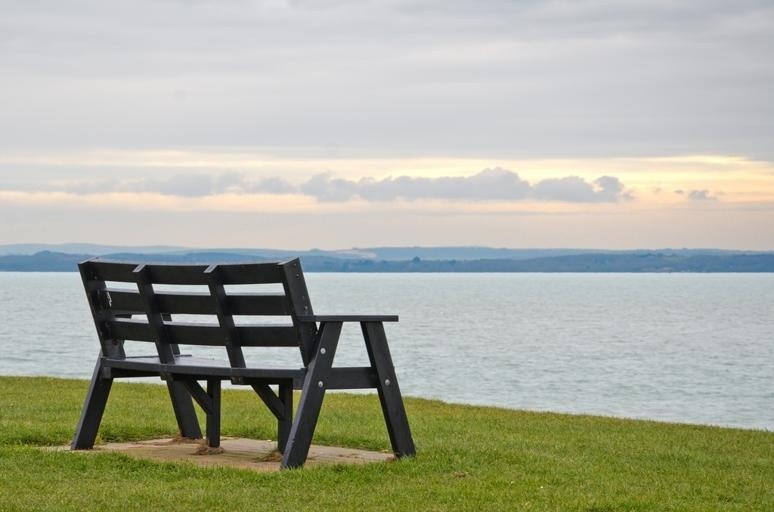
[71,256,416,471]
